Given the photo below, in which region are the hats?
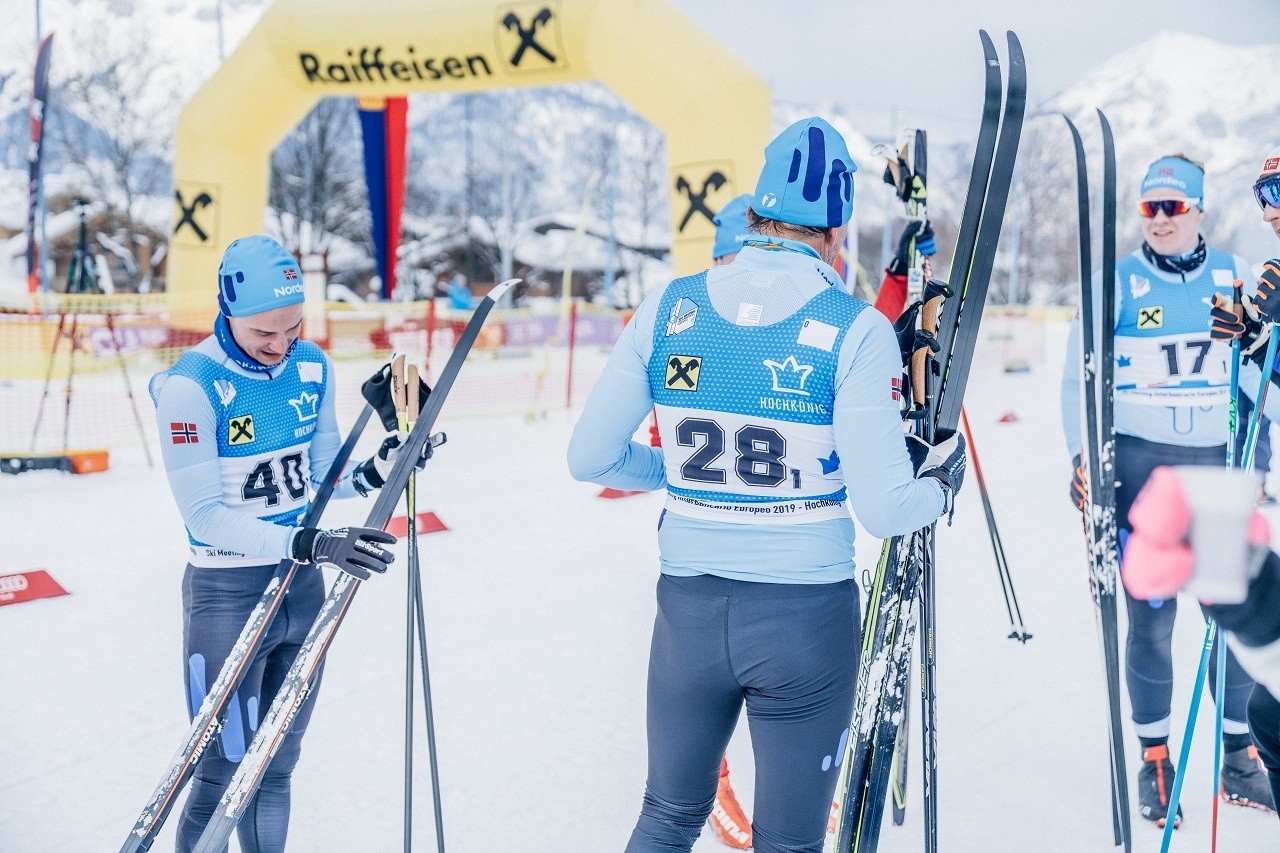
[751,116,857,227]
[217,235,305,317]
[1258,147,1280,178]
[711,195,755,257]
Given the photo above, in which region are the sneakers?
[1220,745,1278,814]
[1137,744,1184,829]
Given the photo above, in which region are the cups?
[1176,466,1264,604]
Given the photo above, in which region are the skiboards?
[1063,107,1136,853]
[120,274,527,852]
[832,22,1033,853]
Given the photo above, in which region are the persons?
[1060,145,1280,830]
[148,233,447,853]
[567,115,966,853]
[448,273,474,311]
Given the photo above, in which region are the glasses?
[1138,197,1202,217]
[1252,172,1280,210]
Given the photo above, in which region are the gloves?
[372,431,446,481]
[1070,453,1123,512]
[896,220,936,264]
[906,429,966,518]
[1119,459,1280,650]
[1252,259,1280,326]
[1210,294,1262,352]
[311,526,397,580]
